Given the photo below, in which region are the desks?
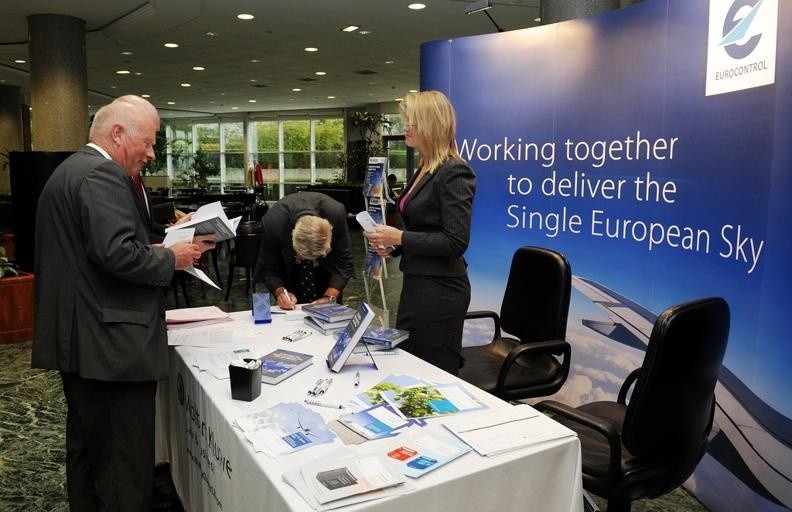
[153,297,584,511]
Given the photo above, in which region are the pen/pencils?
[285,289,294,311]
[366,351,398,355]
[282,331,313,342]
[301,400,345,411]
[307,378,332,397]
[355,371,360,386]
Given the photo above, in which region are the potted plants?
[0,246,36,343]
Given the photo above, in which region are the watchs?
[321,293,338,304]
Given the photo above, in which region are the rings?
[373,238,377,243]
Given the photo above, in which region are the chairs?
[223,223,262,304]
[531,294,731,512]
[453,244,572,400]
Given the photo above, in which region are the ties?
[132,171,149,217]
[300,259,318,303]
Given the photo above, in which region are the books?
[364,157,390,283]
[327,302,375,372]
[301,302,410,354]
[571,276,792,509]
[258,349,315,386]
[165,200,243,244]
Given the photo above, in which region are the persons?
[254,191,358,310]
[30,100,203,512]
[361,89,477,376]
[385,174,399,213]
[83,91,218,255]
